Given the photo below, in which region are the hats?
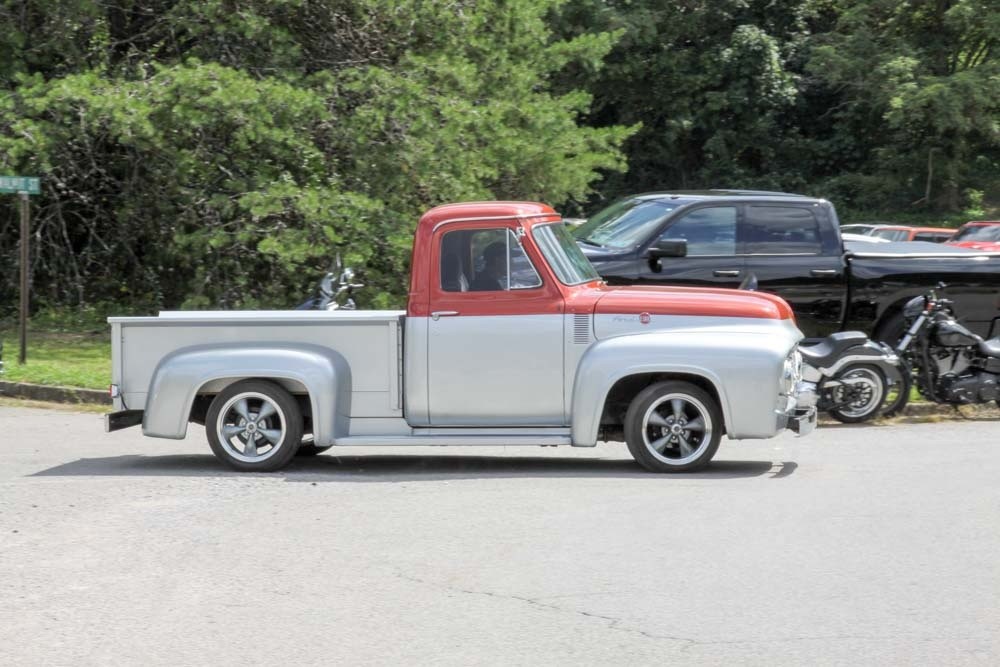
[483,241,522,257]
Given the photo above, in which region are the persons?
[467,240,513,291]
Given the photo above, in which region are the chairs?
[447,257,470,291]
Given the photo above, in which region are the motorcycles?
[877,282,1000,419]
[737,272,902,423]
[296,253,364,311]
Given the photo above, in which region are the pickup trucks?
[510,188,1000,348]
[105,200,818,473]
